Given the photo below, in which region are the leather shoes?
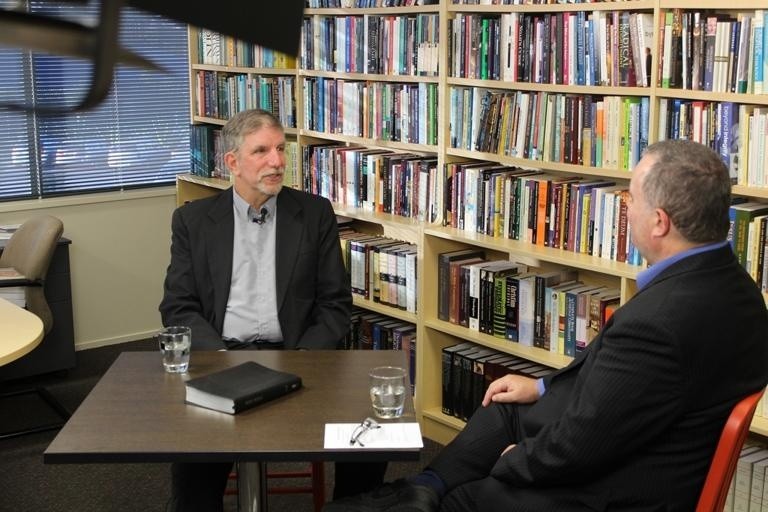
[321,480,435,512]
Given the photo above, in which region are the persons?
[321,139,767,512]
[159,107,353,351]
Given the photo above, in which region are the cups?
[369,365,408,419]
[158,327,191,374]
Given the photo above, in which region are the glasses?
[349,417,382,448]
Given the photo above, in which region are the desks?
[43,350,424,512]
[0,294,44,367]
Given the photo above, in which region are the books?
[299,141,437,220]
[655,97,768,186]
[449,86,648,172]
[185,360,303,415]
[339,314,416,389]
[196,15,438,75]
[723,390,768,511]
[309,0,615,7]
[421,246,622,422]
[191,71,296,125]
[725,201,768,291]
[333,223,416,313]
[449,16,652,84]
[189,124,296,184]
[657,8,768,94]
[444,160,642,264]
[303,78,438,142]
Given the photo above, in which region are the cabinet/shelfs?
[0,236,78,383]
[420,1,768,512]
[176,0,442,422]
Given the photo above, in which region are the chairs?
[695,387,768,511]
[0,214,72,440]
